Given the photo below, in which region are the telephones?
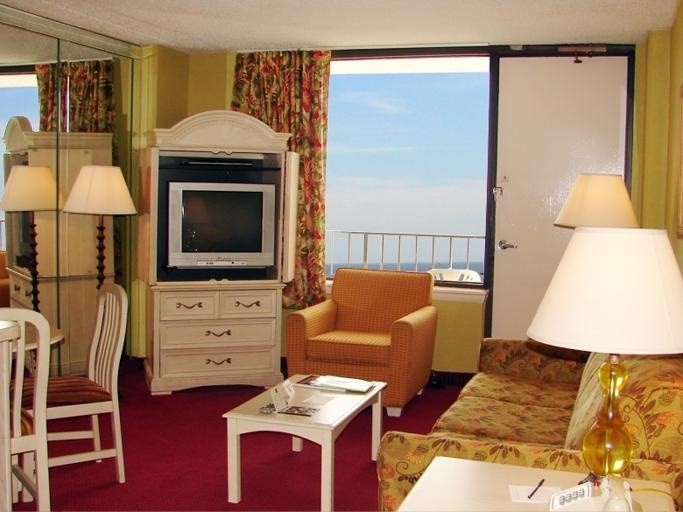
[549,475,636,512]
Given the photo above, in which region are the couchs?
[377,338,683,512]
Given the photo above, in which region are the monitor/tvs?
[167,182,275,270]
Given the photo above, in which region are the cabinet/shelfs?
[139,110,294,395]
[1,117,113,377]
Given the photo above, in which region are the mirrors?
[0,4,142,378]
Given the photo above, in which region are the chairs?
[0,307,51,511]
[10,283,128,483]
[429,268,482,284]
[286,268,437,416]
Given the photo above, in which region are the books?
[294,375,375,393]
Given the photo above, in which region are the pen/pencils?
[527,479,545,498]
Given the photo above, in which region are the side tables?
[393,457,677,512]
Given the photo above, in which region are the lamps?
[554,173,640,229]
[63,165,138,290]
[526,225,683,485]
[0,164,62,313]
